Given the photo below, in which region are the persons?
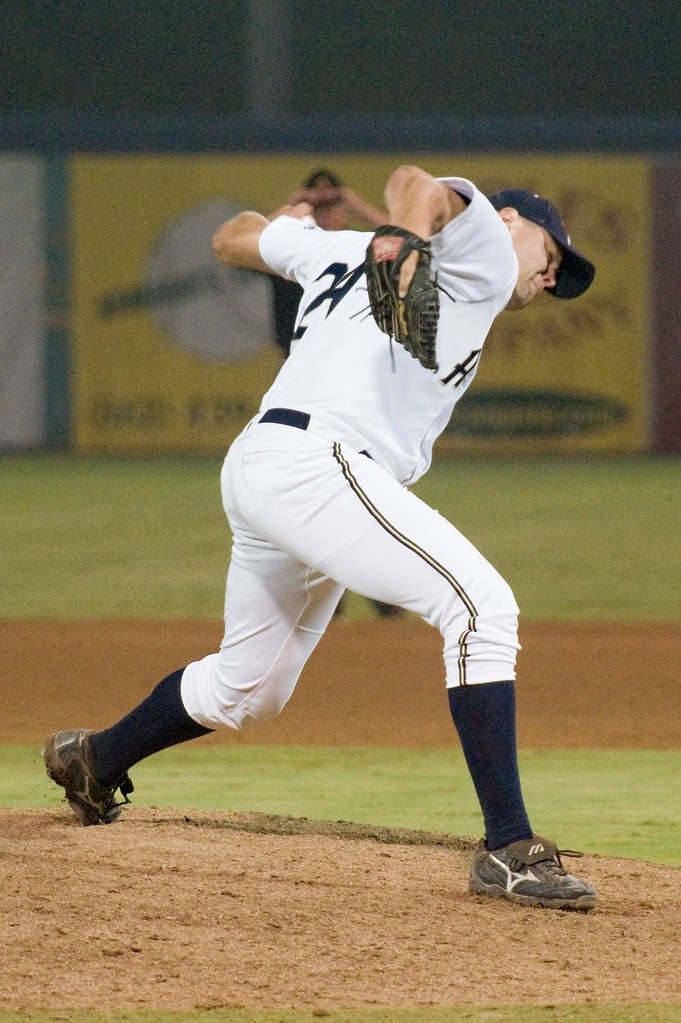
[43,166,598,907]
[272,170,384,356]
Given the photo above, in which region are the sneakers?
[469,833,597,909]
[43,728,134,827]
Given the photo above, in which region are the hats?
[485,189,595,299]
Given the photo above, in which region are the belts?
[258,408,372,459]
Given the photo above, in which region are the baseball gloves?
[364,224,440,371]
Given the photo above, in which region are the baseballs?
[300,213,318,227]
